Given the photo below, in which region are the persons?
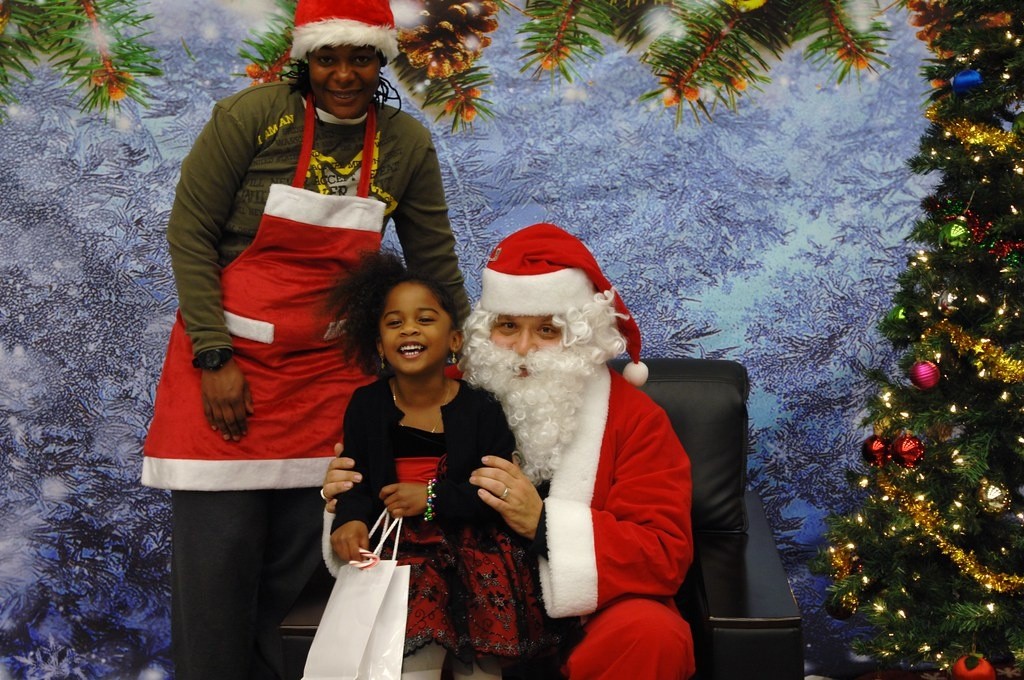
[321,221,697,680]
[328,249,517,680]
[140,0,472,680]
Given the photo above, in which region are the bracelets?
[424,479,437,521]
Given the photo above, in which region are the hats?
[290,0,400,60]
[480,221,649,388]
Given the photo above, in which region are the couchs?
[278,358,806,680]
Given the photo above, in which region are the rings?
[500,488,508,499]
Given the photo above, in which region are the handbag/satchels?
[298,508,406,680]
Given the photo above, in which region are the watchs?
[193,348,232,371]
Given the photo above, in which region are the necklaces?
[393,379,450,434]
